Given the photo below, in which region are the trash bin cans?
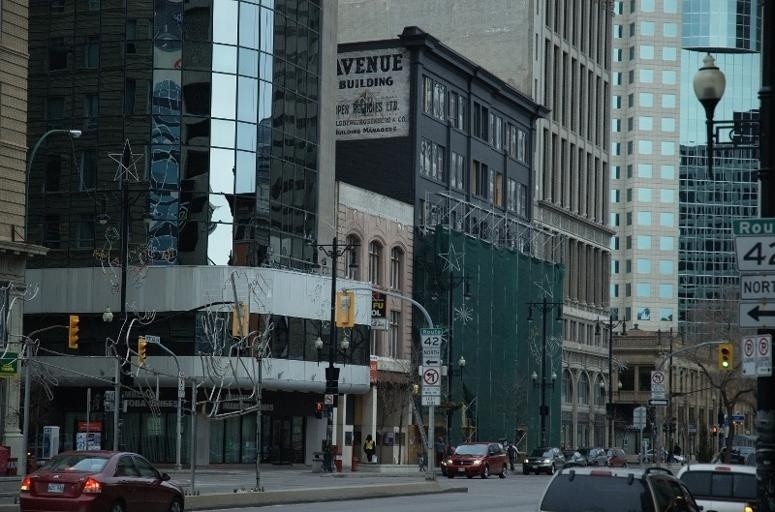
[311,458,322,473]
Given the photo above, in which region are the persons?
[435,436,447,468]
[505,442,521,471]
[362,433,376,462]
[673,441,681,463]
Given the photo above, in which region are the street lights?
[314,235,361,469]
[594,313,628,446]
[24,127,84,245]
[526,298,565,446]
[427,266,472,453]
[92,179,157,456]
[681,0,774,511]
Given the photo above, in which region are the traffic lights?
[315,403,323,411]
[718,343,733,372]
[68,315,80,350]
[138,336,147,363]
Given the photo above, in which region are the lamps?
[693,49,761,182]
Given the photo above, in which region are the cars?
[16,449,185,511]
